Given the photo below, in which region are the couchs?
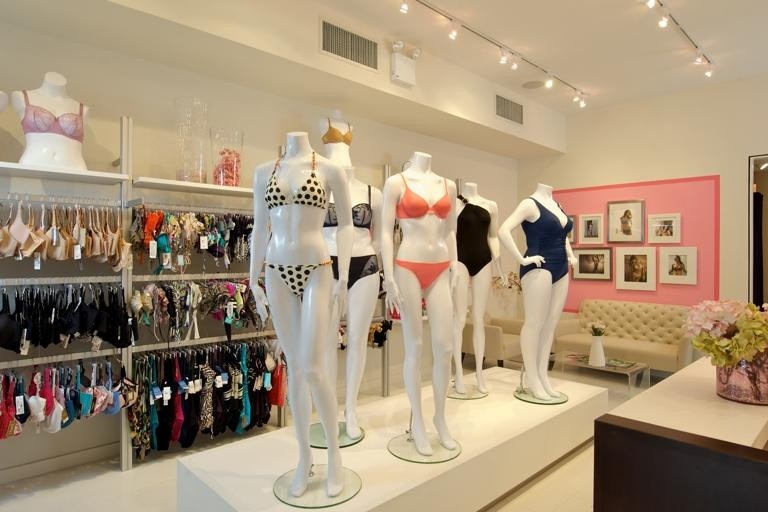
[549,298,693,373]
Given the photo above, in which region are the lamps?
[646,0,716,78]
[399,0,589,108]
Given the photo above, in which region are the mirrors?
[748,154,768,311]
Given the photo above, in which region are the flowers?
[682,299,768,368]
[587,320,605,336]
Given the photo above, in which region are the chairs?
[462,306,525,367]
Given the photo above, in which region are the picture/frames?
[648,213,681,244]
[579,214,603,245]
[572,247,612,281]
[566,215,576,243]
[607,199,645,244]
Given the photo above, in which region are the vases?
[716,348,768,405]
[589,336,605,367]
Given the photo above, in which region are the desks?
[593,351,768,512]
[177,366,608,512]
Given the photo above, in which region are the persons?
[587,220,595,238]
[11,70,90,171]
[620,209,632,237]
[320,167,383,441]
[669,255,687,275]
[321,109,354,171]
[248,132,356,498]
[452,182,500,396]
[380,151,460,456]
[497,182,579,401]
[592,254,600,274]
[629,256,645,282]
[657,221,672,237]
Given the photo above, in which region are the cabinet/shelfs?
[0,160,390,470]
